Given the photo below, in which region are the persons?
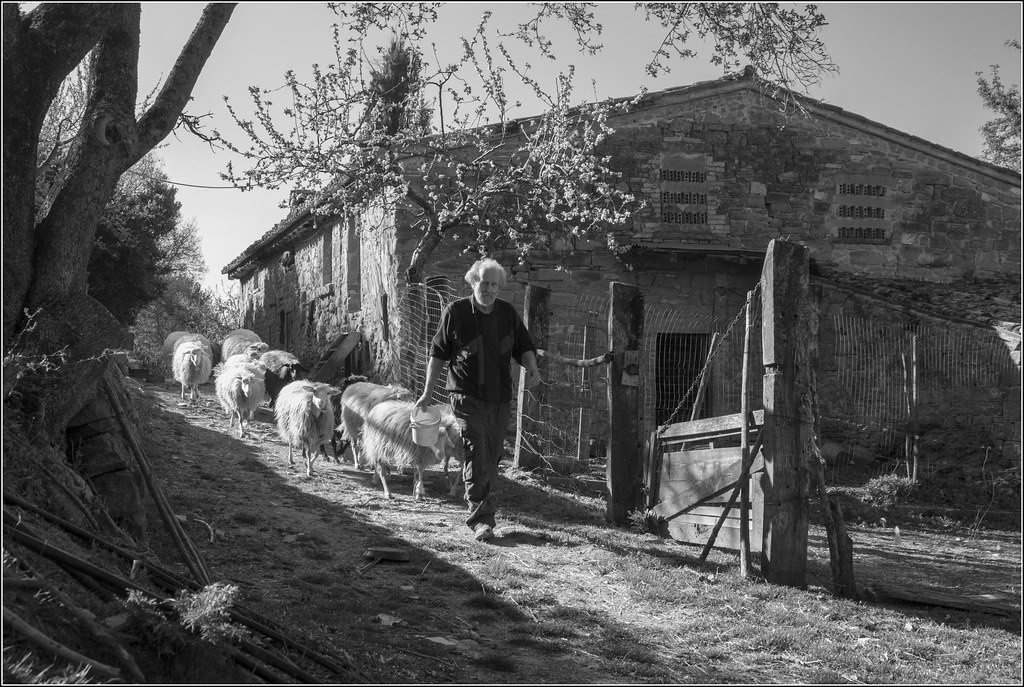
[416,258,541,539]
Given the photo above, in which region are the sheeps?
[333,381,418,471]
[274,375,368,477]
[357,398,474,503]
[210,329,311,438]
[161,331,212,403]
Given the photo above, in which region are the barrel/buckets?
[820,442,848,466]
[410,404,442,445]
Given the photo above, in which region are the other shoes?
[475,523,494,541]
